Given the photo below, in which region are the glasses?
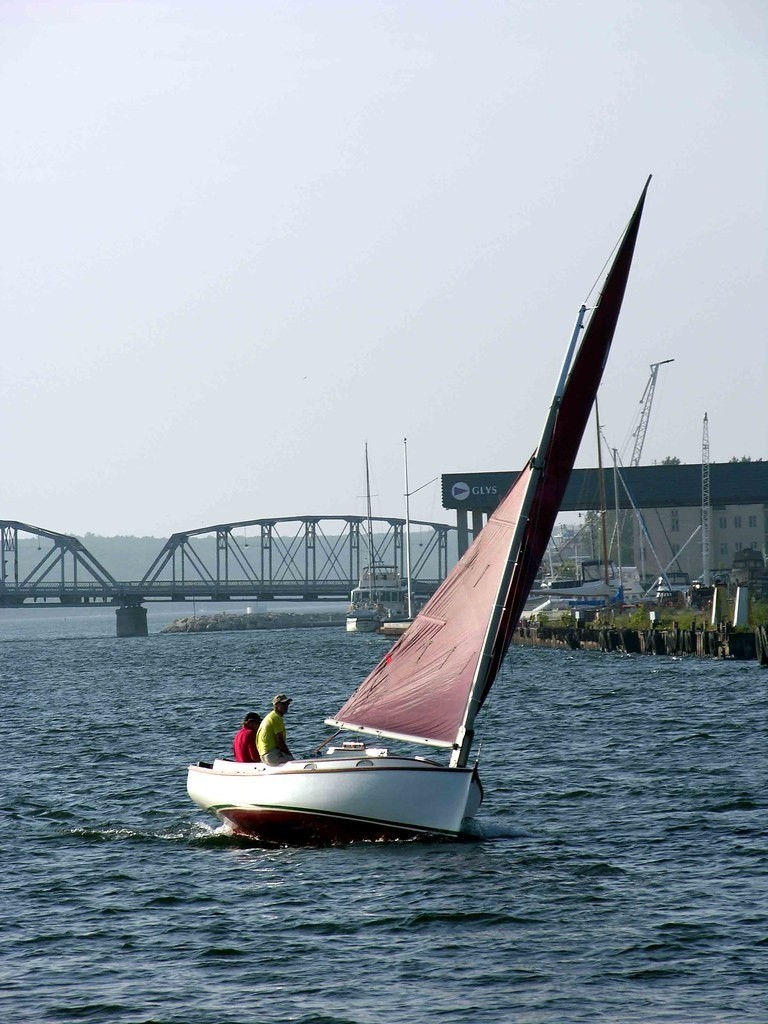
[279,702,290,705]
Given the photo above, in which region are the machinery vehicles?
[568,358,719,608]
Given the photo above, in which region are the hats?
[273,695,292,705]
[246,713,263,721]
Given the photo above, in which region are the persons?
[233,712,262,762]
[256,694,295,766]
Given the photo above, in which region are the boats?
[514,582,610,623]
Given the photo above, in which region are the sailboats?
[180,169,654,837]
[345,439,416,633]
